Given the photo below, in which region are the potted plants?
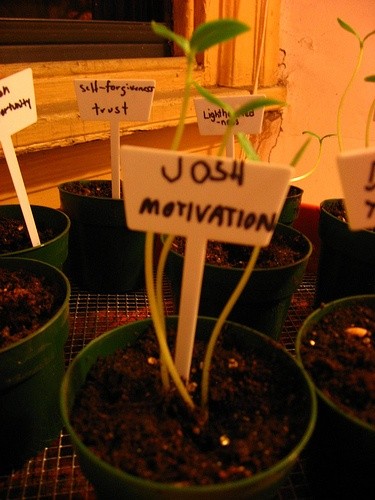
[57,19,375,499]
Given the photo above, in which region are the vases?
[0,204,72,468]
[296,295,375,473]
